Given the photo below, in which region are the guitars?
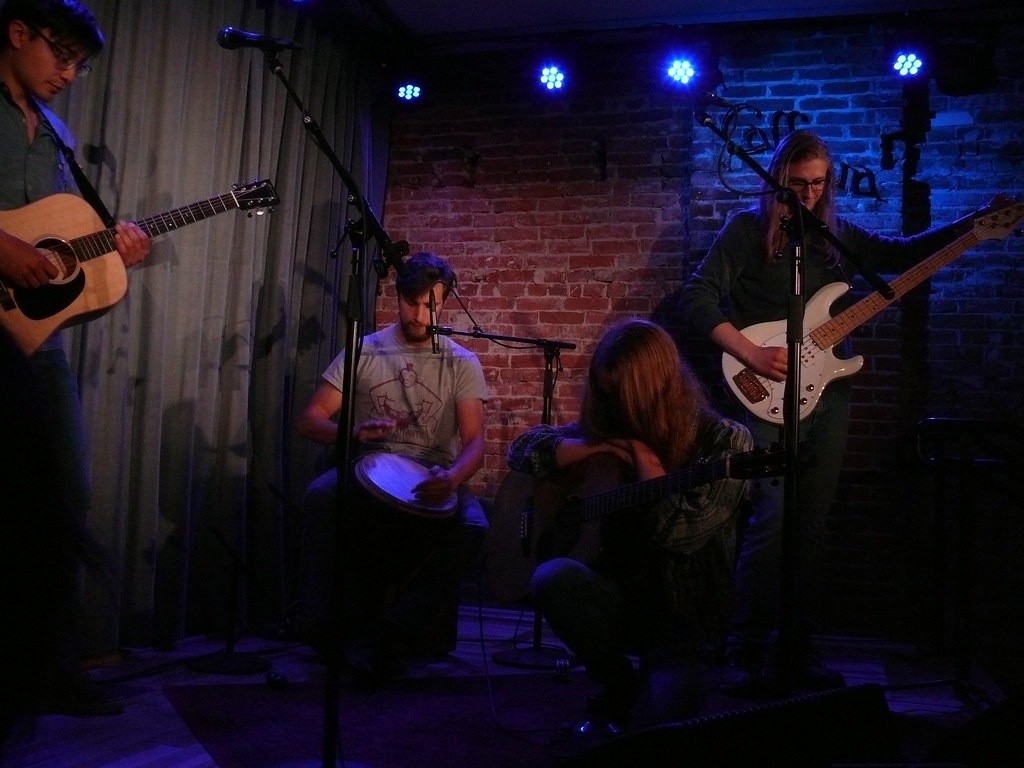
[717,196,1024,430]
[482,441,790,612]
[0,175,284,364]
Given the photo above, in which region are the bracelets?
[975,209,982,217]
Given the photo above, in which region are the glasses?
[789,177,828,191]
[29,26,93,83]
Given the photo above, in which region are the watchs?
[986,202,995,212]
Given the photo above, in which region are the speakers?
[560,681,1024,768]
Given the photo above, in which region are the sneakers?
[49,665,128,716]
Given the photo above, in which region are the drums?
[344,450,459,591]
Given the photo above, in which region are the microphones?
[216,26,304,51]
[660,75,730,107]
[428,288,440,354]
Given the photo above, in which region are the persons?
[505,320,755,722]
[683,128,1014,696]
[296,251,489,647]
[0,0,150,716]
